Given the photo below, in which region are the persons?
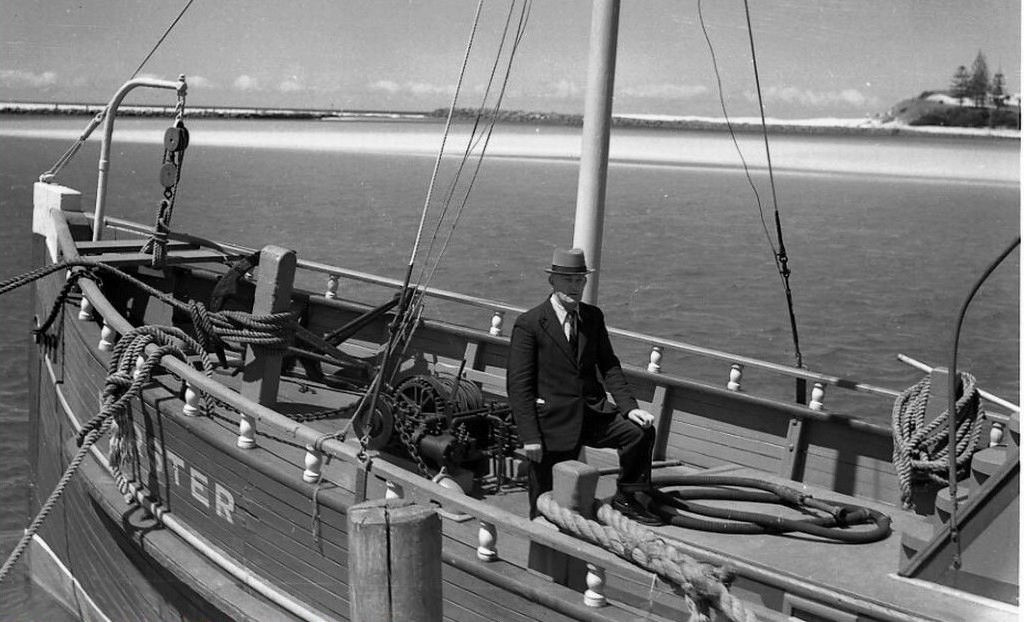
[507,249,663,589]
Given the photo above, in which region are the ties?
[567,311,578,362]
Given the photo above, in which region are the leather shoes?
[610,498,664,526]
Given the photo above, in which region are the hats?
[545,249,597,275]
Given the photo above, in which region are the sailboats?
[23,5,1018,621]
